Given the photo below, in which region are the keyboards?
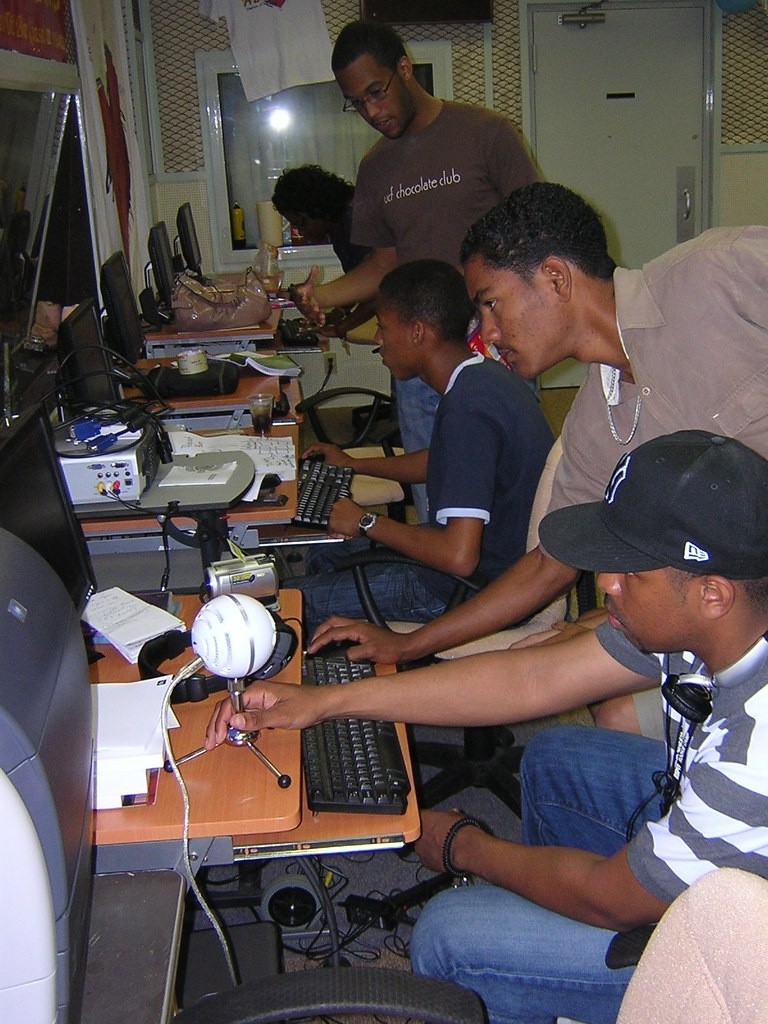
[290,458,353,530]
[272,391,290,417]
[299,647,412,816]
[279,318,320,348]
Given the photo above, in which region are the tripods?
[163,675,291,789]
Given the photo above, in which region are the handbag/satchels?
[170,265,273,332]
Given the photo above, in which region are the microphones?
[179,592,277,682]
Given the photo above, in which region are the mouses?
[299,454,326,465]
[304,639,360,661]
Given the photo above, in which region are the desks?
[64,312,424,1024]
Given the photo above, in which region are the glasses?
[342,65,397,113]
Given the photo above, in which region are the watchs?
[359,511,383,538]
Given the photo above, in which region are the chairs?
[333,541,524,821]
[294,375,417,526]
[167,867,768,1024]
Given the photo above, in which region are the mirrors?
[216,63,434,252]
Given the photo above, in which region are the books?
[158,461,237,487]
[91,675,180,809]
[215,353,301,376]
[81,591,184,645]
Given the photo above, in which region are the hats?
[538,429,768,581]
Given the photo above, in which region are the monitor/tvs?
[174,202,203,277]
[59,298,118,422]
[99,250,147,369]
[0,402,108,666]
[144,220,174,309]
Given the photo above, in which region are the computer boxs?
[50,415,162,505]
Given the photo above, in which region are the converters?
[346,893,400,930]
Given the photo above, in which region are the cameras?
[200,552,283,612]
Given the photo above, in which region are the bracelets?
[442,817,481,877]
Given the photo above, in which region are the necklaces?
[607,366,641,446]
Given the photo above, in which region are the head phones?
[661,628,768,722]
[138,608,299,704]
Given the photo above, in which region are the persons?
[271,165,376,338]
[302,261,557,648]
[308,183,768,743]
[295,18,547,525]
[203,430,768,1024]
